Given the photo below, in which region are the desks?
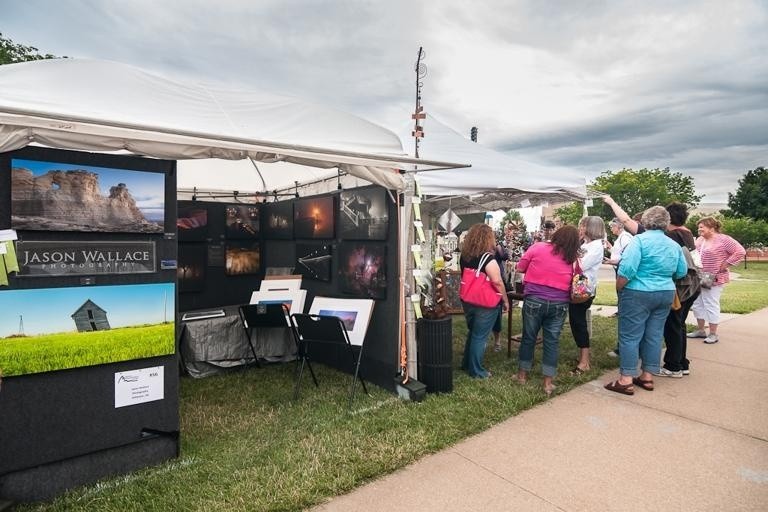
[504,284,594,359]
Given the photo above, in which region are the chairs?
[289,309,369,403]
[236,304,319,393]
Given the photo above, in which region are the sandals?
[632,376,655,392]
[603,380,634,396]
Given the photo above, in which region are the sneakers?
[511,375,527,385]
[607,351,617,358]
[544,384,556,395]
[653,366,691,379]
[567,358,591,376]
[493,344,502,351]
[686,328,719,344]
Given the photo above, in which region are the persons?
[685,215,746,344]
[457,222,512,378]
[510,225,579,394]
[603,205,687,394]
[542,213,588,244]
[567,214,605,376]
[633,212,644,225]
[602,216,632,318]
[489,242,509,353]
[602,192,703,377]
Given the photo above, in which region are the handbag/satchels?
[697,270,716,289]
[459,267,503,309]
[570,273,595,304]
[671,289,682,311]
